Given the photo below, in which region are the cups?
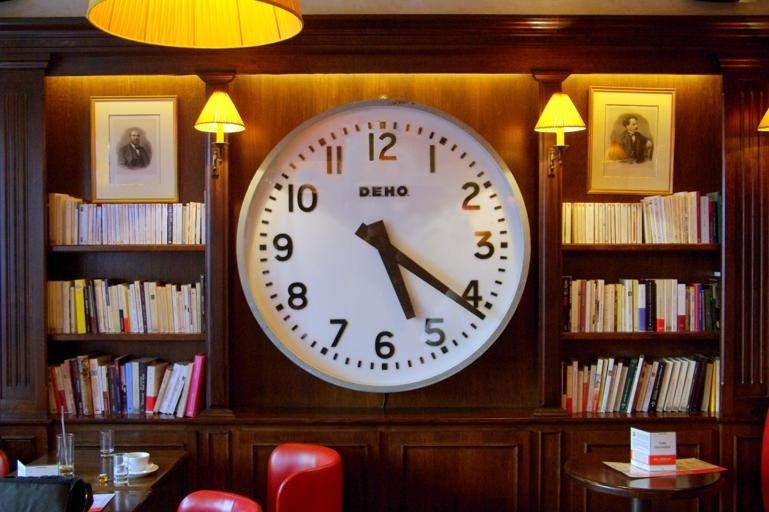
[110,453,128,486]
[123,450,149,470]
[56,433,75,475]
[99,429,114,456]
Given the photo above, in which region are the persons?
[610,115,655,166]
[116,129,151,170]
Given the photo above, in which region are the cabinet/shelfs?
[539,15,769,413]
[6,17,204,420]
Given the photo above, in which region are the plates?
[123,463,160,478]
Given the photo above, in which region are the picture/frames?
[588,85,676,197]
[89,94,181,202]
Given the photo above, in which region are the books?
[46,192,206,419]
[561,190,720,417]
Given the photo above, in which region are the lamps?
[194,91,247,181]
[86,2,303,51]
[757,108,769,133]
[533,94,587,177]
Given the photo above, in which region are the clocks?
[235,98,534,394]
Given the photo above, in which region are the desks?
[563,453,724,511]
[5,449,185,512]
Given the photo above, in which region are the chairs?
[267,441,343,510]
[178,489,260,510]
[0,450,10,475]
